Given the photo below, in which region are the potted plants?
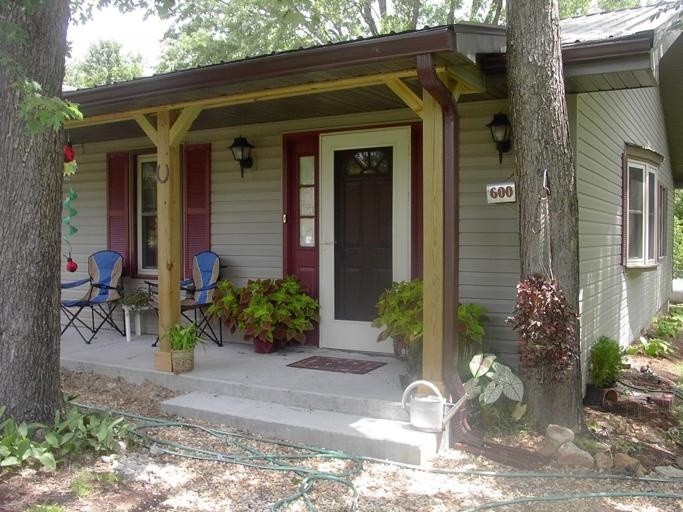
[372,276,490,360]
[208,272,320,354]
[582,332,626,410]
[159,317,210,374]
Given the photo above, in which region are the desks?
[121,298,154,343]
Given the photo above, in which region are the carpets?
[284,353,387,376]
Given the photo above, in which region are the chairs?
[57,250,127,344]
[141,249,224,348]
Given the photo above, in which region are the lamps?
[483,110,515,166]
[225,134,256,180]
